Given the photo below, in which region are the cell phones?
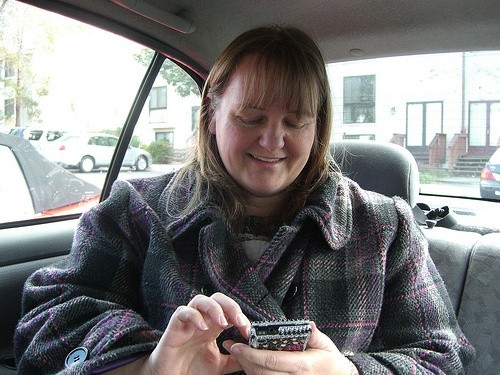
[248,318,312,351]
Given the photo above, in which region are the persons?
[13,24,476,375]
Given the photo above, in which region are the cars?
[23,127,65,152]
[0,132,102,226]
[49,132,151,172]
[480,147,500,199]
[9,127,26,136]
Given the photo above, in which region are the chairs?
[329,141,500,375]
